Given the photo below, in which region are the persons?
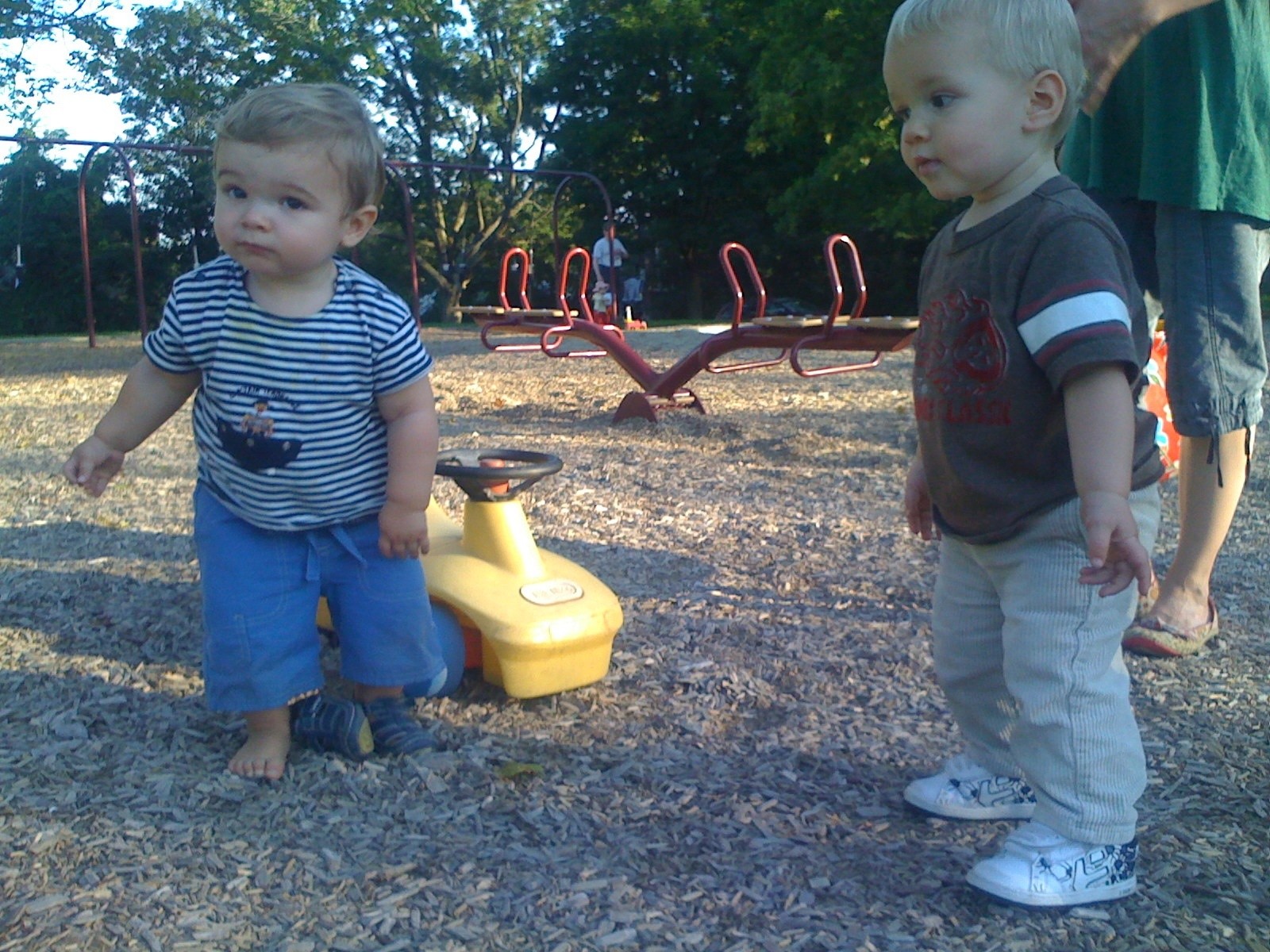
[884,0,1168,904]
[1054,0,1270,658]
[592,223,643,325]
[65,80,446,779]
